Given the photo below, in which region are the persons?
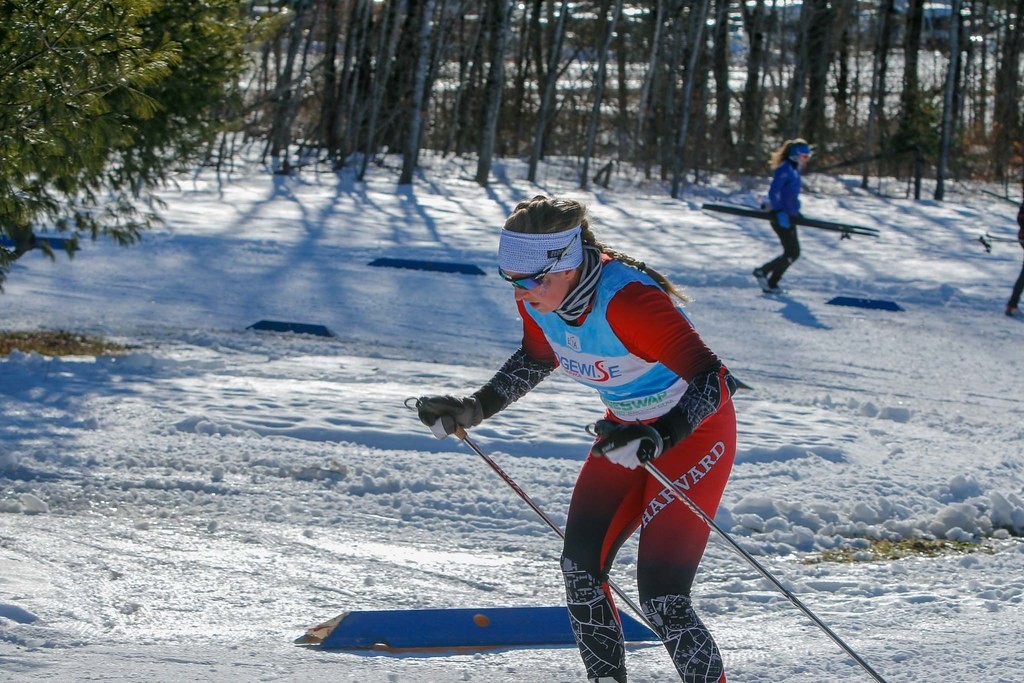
[419,195,754,683]
[1005,179,1024,316]
[752,139,811,293]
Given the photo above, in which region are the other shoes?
[753,268,770,291]
[763,285,788,294]
[1005,305,1016,316]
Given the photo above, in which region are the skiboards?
[700,202,882,239]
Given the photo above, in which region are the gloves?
[591,406,692,469]
[777,212,790,228]
[416,395,484,440]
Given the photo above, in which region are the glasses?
[498,233,576,290]
[798,154,810,161]
